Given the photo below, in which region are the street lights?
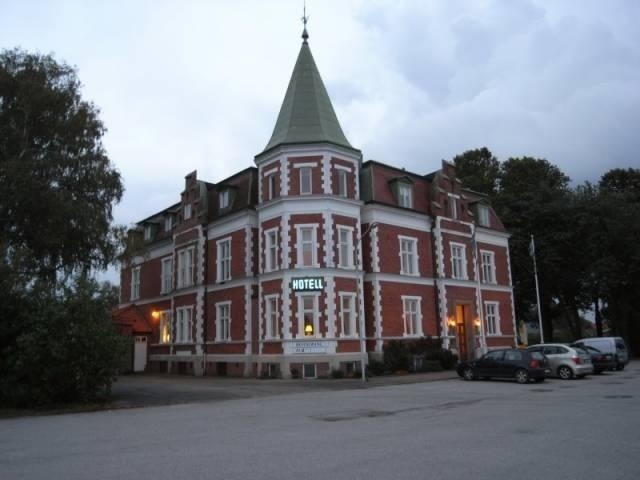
[355,220,380,385]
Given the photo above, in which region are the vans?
[571,337,630,371]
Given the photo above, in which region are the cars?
[456,348,551,384]
[521,342,594,380]
[568,345,615,376]
